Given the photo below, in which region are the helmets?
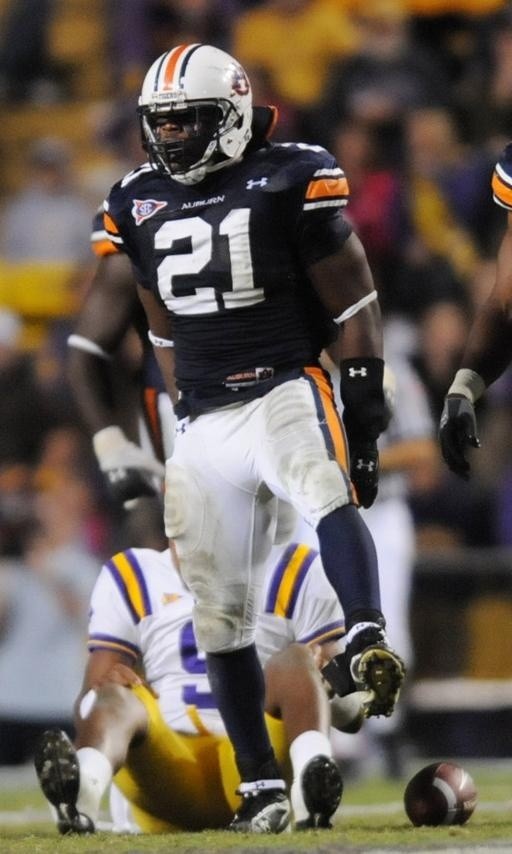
[137,43,253,186]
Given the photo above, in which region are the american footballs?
[404,762,478,826]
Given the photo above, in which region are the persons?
[35,548,372,831]
[1,0,510,770]
[66,41,404,831]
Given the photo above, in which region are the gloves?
[438,393,481,481]
[346,431,379,509]
[92,425,157,503]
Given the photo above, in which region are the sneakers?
[33,728,103,835]
[225,746,344,834]
[345,608,406,719]
